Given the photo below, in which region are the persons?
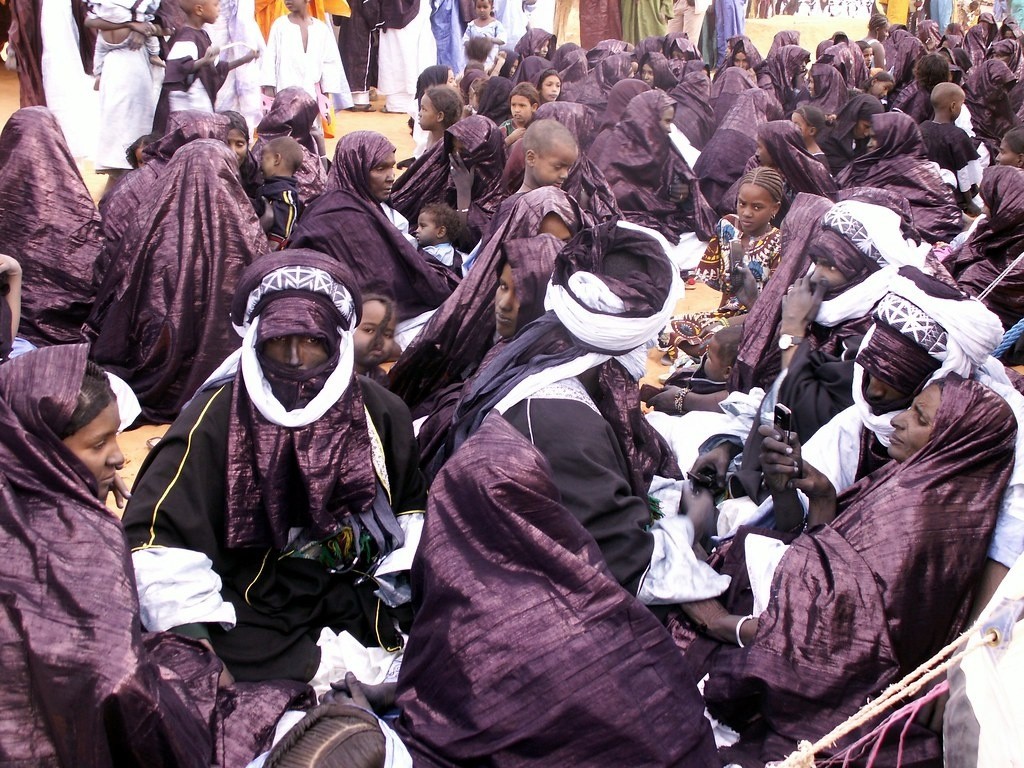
[99,109,229,284]
[121,138,264,411]
[5,1,95,159]
[664,371,1018,747]
[450,213,765,650]
[719,266,1024,731]
[83,2,164,198]
[643,1,1024,415]
[121,245,427,690]
[0,103,89,333]
[297,128,424,314]
[91,1,166,88]
[351,293,399,384]
[0,253,38,363]
[242,702,456,768]
[497,184,586,245]
[203,0,679,224]
[127,134,164,169]
[492,235,575,344]
[416,204,472,282]
[154,1,261,133]
[1,341,224,767]
[318,405,672,767]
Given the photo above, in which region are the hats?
[809,201,904,278]
[231,246,363,356]
[545,220,688,356]
[853,265,1003,395]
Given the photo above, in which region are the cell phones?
[639,384,662,404]
[773,403,792,444]
[729,238,745,287]
[688,470,716,487]
[145,437,161,449]
